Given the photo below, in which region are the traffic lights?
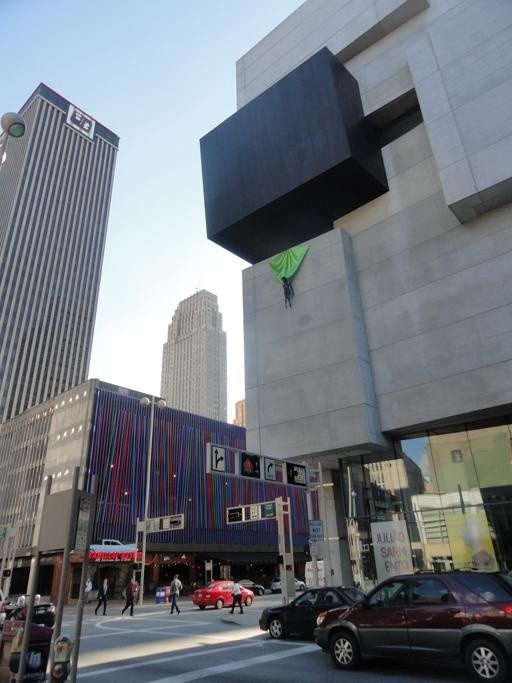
[170,520,179,524]
[228,512,239,519]
[0,110,25,137]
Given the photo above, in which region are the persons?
[120,576,138,616]
[230,577,244,613]
[169,572,183,614]
[85,575,93,604]
[95,576,110,615]
[281,276,295,309]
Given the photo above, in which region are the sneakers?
[95,609,106,615]
[171,610,180,615]
[121,609,133,616]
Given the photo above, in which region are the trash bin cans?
[156,587,166,604]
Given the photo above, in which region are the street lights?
[139,395,167,608]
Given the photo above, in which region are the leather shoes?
[229,611,243,614]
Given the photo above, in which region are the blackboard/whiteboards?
[371,519,414,584]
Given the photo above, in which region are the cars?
[3,592,55,628]
[269,576,304,592]
[311,568,512,683]
[192,579,265,608]
[257,585,368,640]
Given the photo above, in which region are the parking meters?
[50,634,73,682]
